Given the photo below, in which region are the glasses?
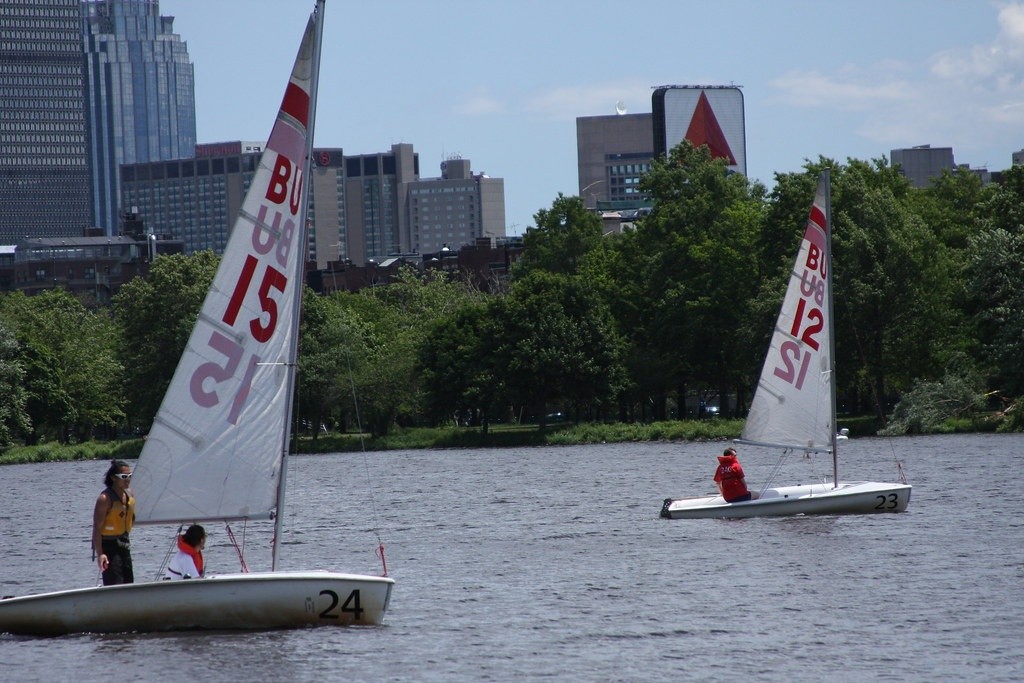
[112,472,133,479]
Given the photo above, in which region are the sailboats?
[0,1,397,641]
[658,166,912,521]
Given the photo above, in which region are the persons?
[92,460,136,587]
[163,522,206,583]
[713,447,760,503]
